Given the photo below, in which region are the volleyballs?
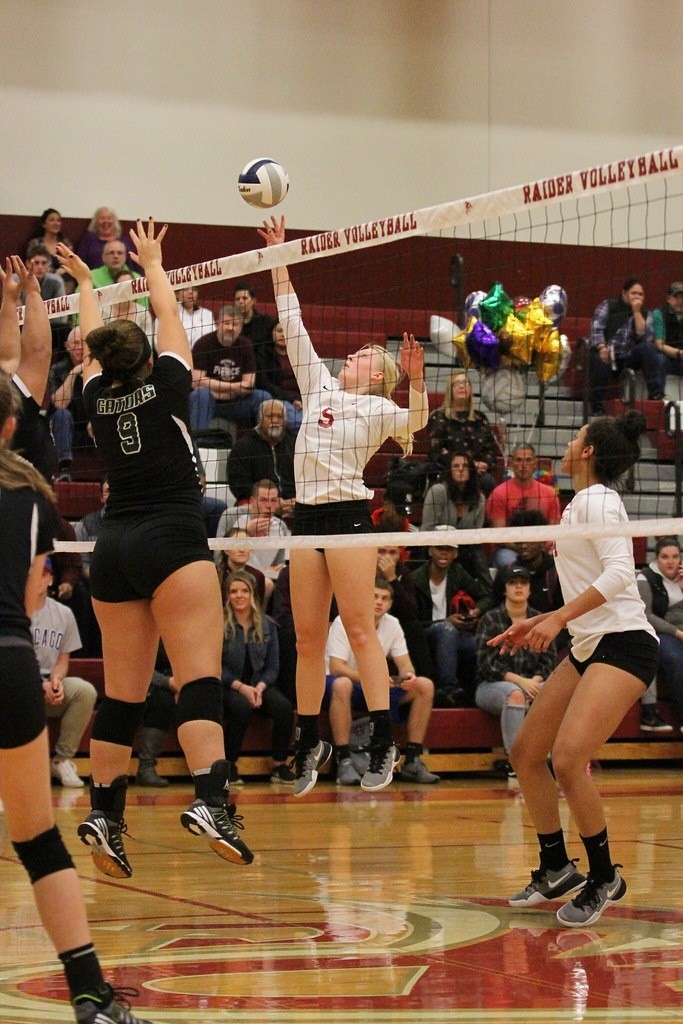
[238,158,290,209]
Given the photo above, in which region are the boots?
[134,727,170,786]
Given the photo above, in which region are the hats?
[433,525,460,549]
[505,567,531,580]
[669,281,683,296]
[387,482,414,517]
[44,559,52,572]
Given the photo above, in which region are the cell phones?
[391,675,408,687]
[463,615,478,621]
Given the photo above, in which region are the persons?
[0,208,683,787]
[487,412,661,928]
[255,215,429,797]
[55,216,257,880]
[0,255,54,625]
[0,370,155,1024]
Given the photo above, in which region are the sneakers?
[555,861,627,928]
[271,763,296,785]
[57,789,84,811]
[229,763,244,785]
[180,797,255,865]
[364,743,400,792]
[75,983,150,1024]
[336,761,362,785]
[52,756,85,788]
[507,858,588,907]
[290,740,334,796]
[402,757,439,784]
[77,809,133,879]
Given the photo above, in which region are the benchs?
[42,296,683,759]
